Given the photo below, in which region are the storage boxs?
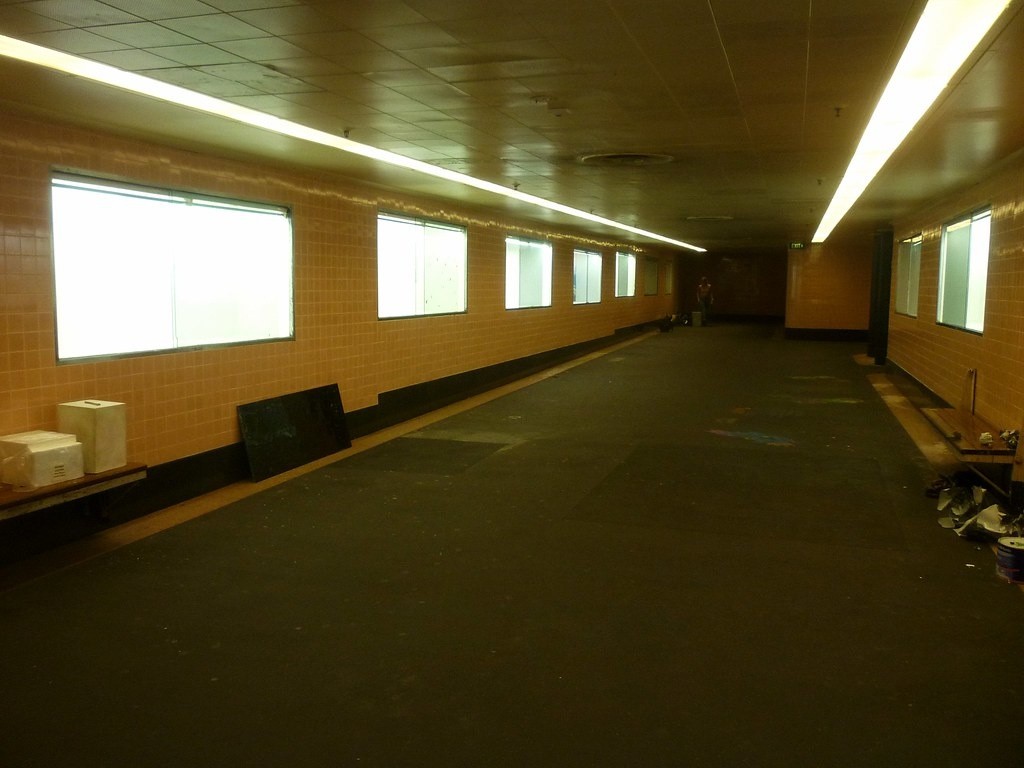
[57,398,128,474]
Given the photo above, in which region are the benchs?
[919,405,1015,464]
[0,461,148,522]
